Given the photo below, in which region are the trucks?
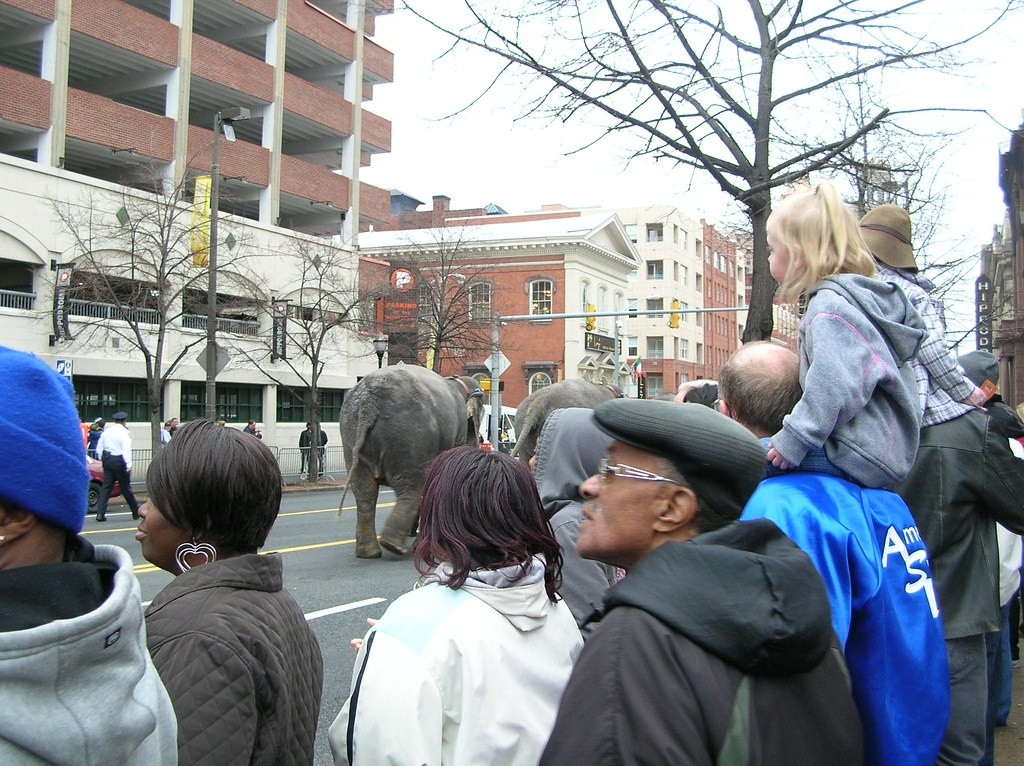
[477,404,517,449]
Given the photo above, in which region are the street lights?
[372,332,389,369]
[202,106,251,421]
[614,295,660,386]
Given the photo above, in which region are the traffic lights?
[498,381,505,393]
[585,304,597,331]
[668,302,681,329]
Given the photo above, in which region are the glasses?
[599,454,683,486]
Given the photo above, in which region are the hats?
[0,345,89,536]
[111,411,128,421]
[858,203,920,274]
[591,397,770,520]
[958,350,1000,388]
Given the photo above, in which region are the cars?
[85,455,123,514]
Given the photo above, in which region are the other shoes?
[96,514,107,521]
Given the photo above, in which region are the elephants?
[511,377,629,469]
[338,361,485,558]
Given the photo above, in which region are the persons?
[649,179,1024,766]
[0,346,180,766]
[242,420,263,440]
[299,420,328,478]
[160,417,180,448]
[87,412,140,522]
[327,397,864,766]
[136,419,324,766]
